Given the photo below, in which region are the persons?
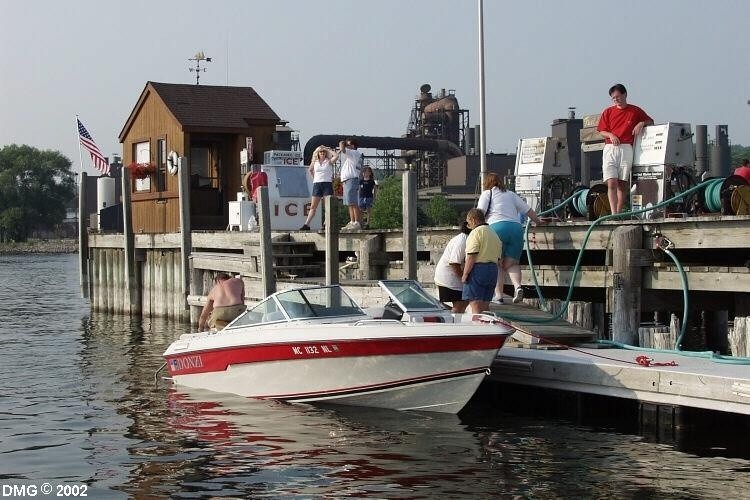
[734,159,750,182]
[299,144,339,230]
[596,83,654,221]
[358,164,379,230]
[198,273,247,333]
[434,222,471,313]
[477,172,542,304]
[460,207,502,313]
[340,137,363,229]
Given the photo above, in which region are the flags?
[77,120,110,175]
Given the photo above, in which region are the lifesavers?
[167,150,177,176]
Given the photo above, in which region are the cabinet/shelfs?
[228,201,255,232]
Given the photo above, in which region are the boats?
[162,280,514,415]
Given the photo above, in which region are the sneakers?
[491,296,504,305]
[300,224,310,230]
[341,223,361,230]
[513,286,524,303]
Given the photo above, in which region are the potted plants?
[128,162,156,179]
